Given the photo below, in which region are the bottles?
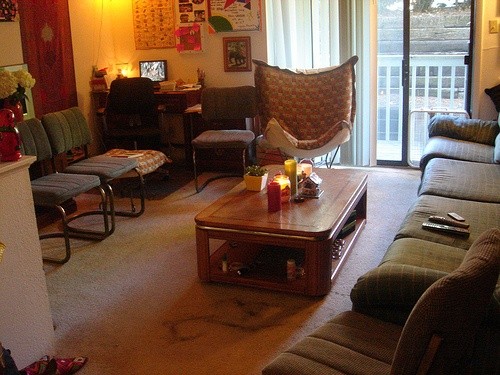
[116,69,122,79]
[287,259,304,282]
[222,255,227,272]
[273,175,291,203]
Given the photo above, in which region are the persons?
[227,46,246,68]
[178,0,205,23]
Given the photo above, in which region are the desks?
[0,155,66,371]
[91,83,202,170]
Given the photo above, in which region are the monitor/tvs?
[139,59,168,82]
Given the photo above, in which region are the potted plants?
[244,164,268,192]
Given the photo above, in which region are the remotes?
[429,216,469,229]
[421,221,470,238]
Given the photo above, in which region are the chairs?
[105,77,162,151]
[42,107,147,234]
[252,57,358,168]
[14,118,111,264]
[190,85,255,193]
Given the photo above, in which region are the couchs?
[348,114,500,320]
[262,227,500,375]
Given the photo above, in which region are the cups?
[297,163,312,184]
[284,159,296,195]
[268,182,281,212]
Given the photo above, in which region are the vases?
[0,107,21,161]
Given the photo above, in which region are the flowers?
[0,65,37,102]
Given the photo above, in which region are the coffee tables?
[193,165,369,296]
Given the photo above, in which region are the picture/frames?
[222,37,252,72]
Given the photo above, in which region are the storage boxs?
[338,210,356,238]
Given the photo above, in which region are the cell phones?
[448,212,465,221]
